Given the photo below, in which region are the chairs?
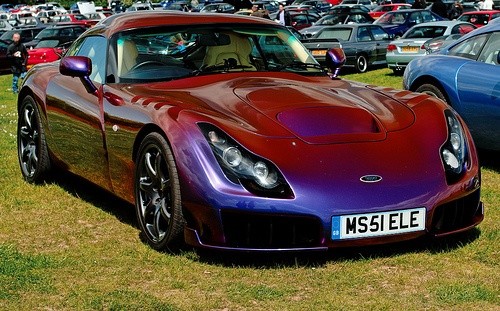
[200,32,257,72]
[118,39,138,73]
[423,29,437,37]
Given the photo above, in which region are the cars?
[359,0,483,40]
[456,11,500,33]
[402,14,500,152]
[0,0,383,73]
[385,18,478,74]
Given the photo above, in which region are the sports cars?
[17,7,485,253]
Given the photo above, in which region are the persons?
[431,0,447,18]
[7,34,28,94]
[448,2,463,34]
[33,7,44,17]
[250,5,262,41]
[263,10,273,45]
[275,4,291,44]
[411,0,423,9]
[478,0,485,9]
